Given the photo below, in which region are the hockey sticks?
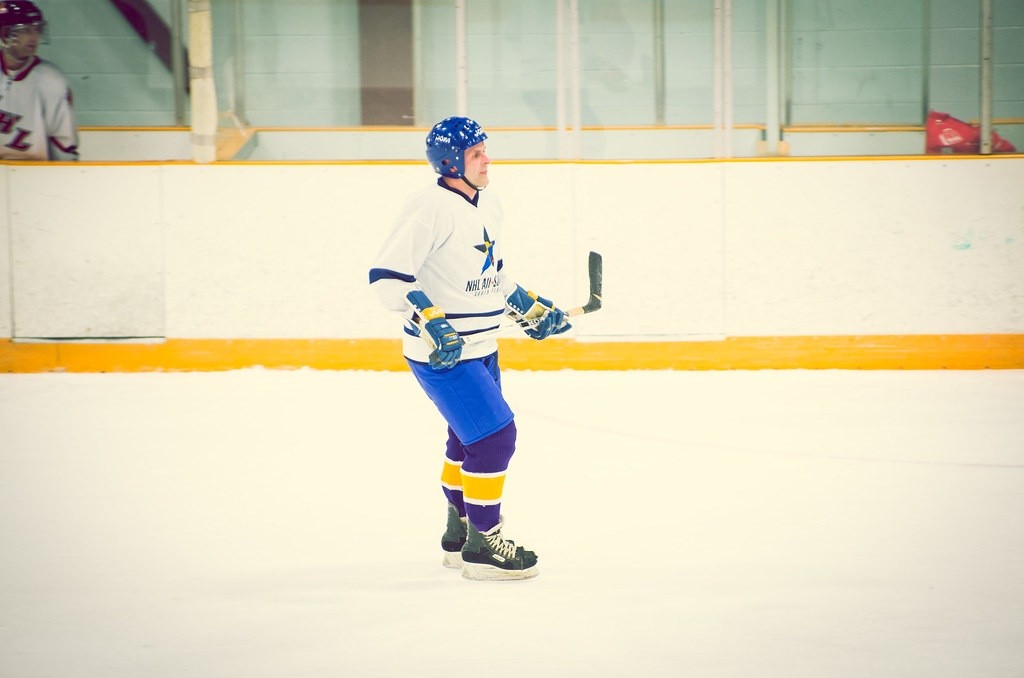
[432,251,602,349]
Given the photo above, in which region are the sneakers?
[440,499,540,581]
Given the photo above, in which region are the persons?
[1,1,81,161]
[370,117,572,582]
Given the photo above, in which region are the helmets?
[424,115,489,179]
[0,0,51,48]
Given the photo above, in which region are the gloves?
[504,283,573,341]
[403,289,463,371]
